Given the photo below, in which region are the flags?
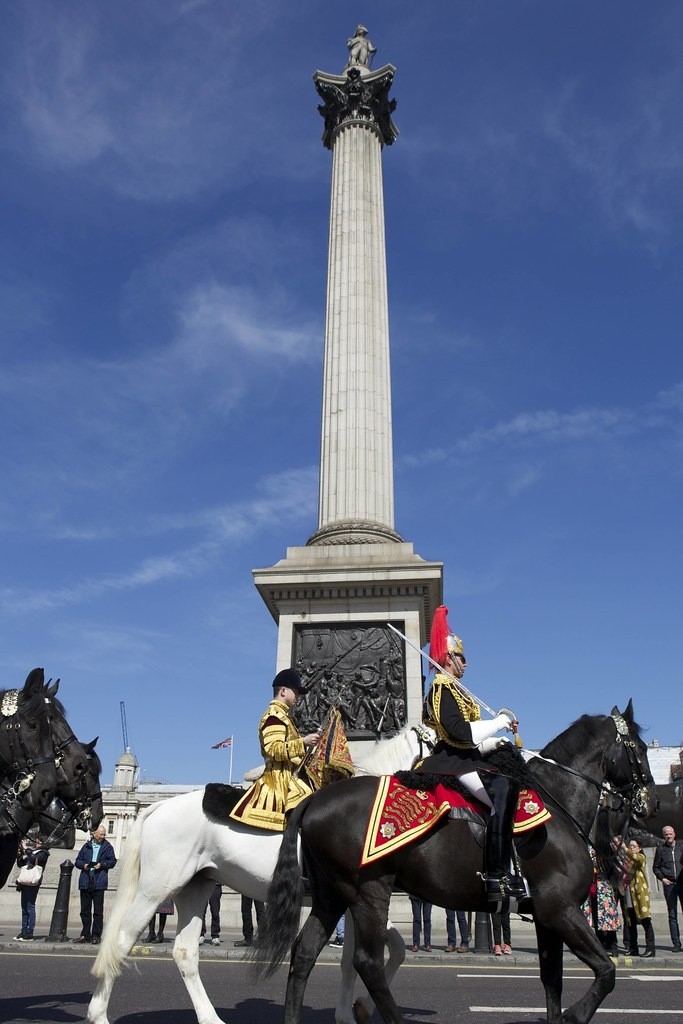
[211,736,231,749]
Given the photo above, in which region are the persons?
[653,826,683,952]
[491,913,512,955]
[142,898,174,943]
[291,656,405,734]
[12,835,50,942]
[580,834,656,958]
[409,895,432,953]
[423,633,527,900]
[197,879,223,946]
[445,909,469,953]
[330,914,345,948]
[235,894,268,947]
[72,825,117,944]
[230,668,319,831]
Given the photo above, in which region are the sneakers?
[504,943,512,954]
[494,943,502,955]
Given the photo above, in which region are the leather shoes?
[641,948,656,958]
[624,949,640,956]
[485,879,541,901]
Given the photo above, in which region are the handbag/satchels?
[18,858,44,886]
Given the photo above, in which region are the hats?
[429,605,467,668]
[270,668,309,694]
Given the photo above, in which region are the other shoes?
[13,934,26,941]
[152,934,163,943]
[610,948,619,958]
[457,945,471,952]
[329,938,344,948]
[211,938,221,947]
[92,937,100,944]
[234,938,250,947]
[424,945,431,951]
[673,946,680,952]
[412,945,419,952]
[199,936,205,945]
[18,934,34,942]
[445,943,456,952]
[142,934,157,944]
[72,935,90,943]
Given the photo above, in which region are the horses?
[252,698,660,1024]
[87,719,440,1024]
[0,668,104,889]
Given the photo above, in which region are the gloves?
[492,712,511,731]
